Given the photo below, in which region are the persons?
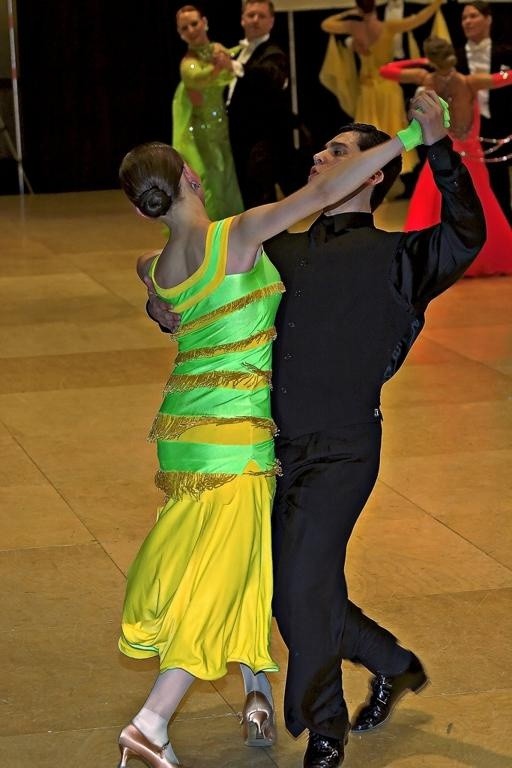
[317,0,512,228]
[379,38,512,277]
[170,0,309,223]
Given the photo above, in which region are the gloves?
[396,97,450,150]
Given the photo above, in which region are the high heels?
[118,721,185,768]
[239,691,276,747]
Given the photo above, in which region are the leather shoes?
[351,649,432,733]
[303,721,352,768]
